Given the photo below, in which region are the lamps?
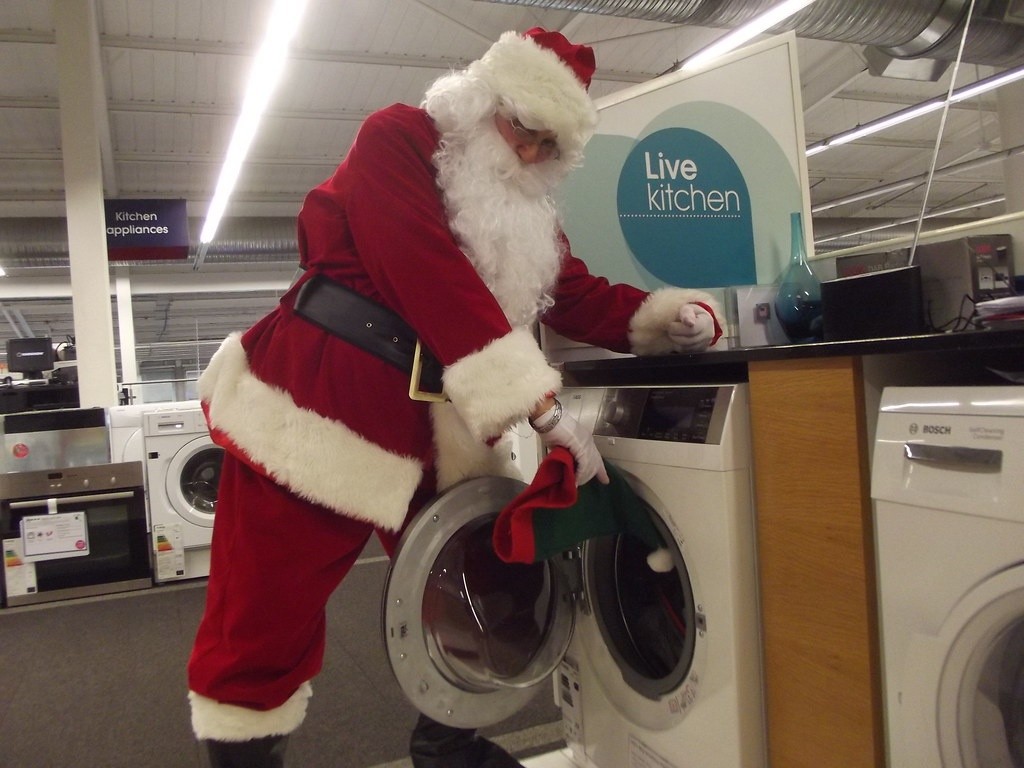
[806,63,1024,161]
[677,0,818,72]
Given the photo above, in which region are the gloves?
[534,405,609,487]
[666,304,715,352]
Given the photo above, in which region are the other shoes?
[206,734,287,768]
[409,709,524,768]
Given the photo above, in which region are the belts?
[288,268,450,403]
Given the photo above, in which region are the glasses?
[508,121,561,162]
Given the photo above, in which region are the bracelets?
[528,397,562,434]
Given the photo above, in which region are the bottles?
[775,212,823,345]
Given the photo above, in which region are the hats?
[479,24,598,150]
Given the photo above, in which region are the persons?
[187,26,724,768]
